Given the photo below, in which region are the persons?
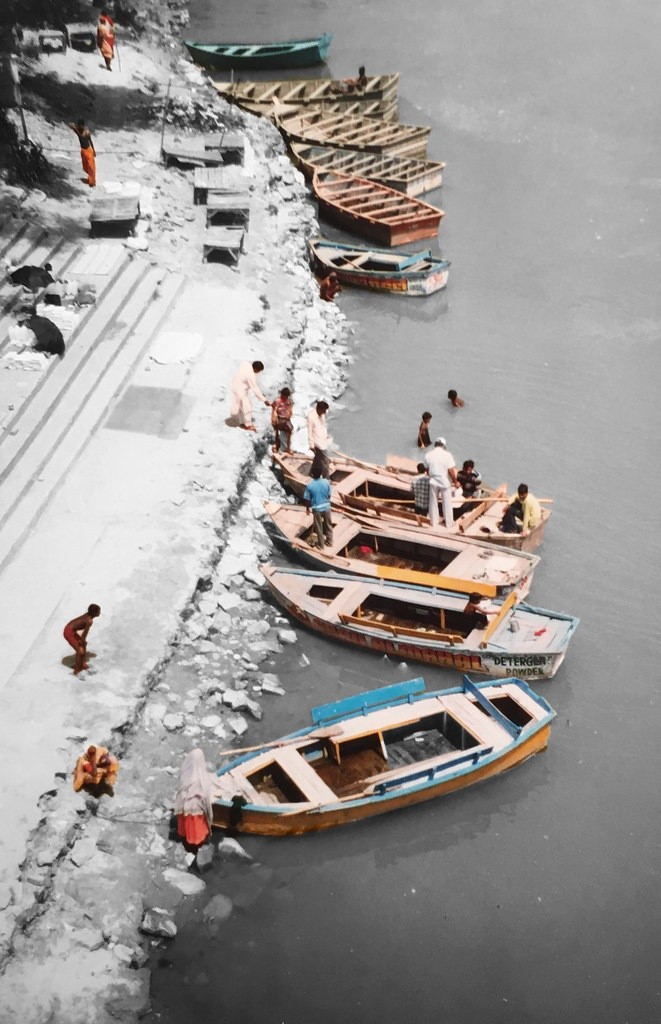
[100,8,114,27]
[410,463,430,517]
[97,16,115,71]
[499,482,542,536]
[462,591,501,635]
[270,388,294,455]
[231,360,271,431]
[448,389,465,408]
[306,400,337,485]
[423,436,461,529]
[457,459,482,498]
[73,740,120,792]
[63,118,97,187]
[320,272,343,302]
[303,462,333,549]
[417,412,433,449]
[328,65,369,94]
[63,604,100,675]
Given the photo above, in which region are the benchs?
[341,250,374,269]
[364,745,493,797]
[314,467,507,580]
[275,750,340,802]
[224,45,261,56]
[439,682,548,750]
[241,75,433,222]
[331,582,517,651]
[395,247,433,273]
[321,698,445,764]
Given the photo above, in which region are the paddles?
[338,254,364,270]
[219,725,344,756]
[331,449,414,484]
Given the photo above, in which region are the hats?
[435,437,447,449]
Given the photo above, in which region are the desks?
[193,167,254,262]
[87,196,142,237]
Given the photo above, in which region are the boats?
[257,563,582,682]
[288,140,448,200]
[271,102,433,158]
[211,673,559,840]
[312,163,447,247]
[304,238,454,298]
[183,30,333,71]
[208,69,402,121]
[273,449,558,553]
[261,499,542,606]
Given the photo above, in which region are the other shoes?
[324,538,333,547]
[241,423,256,430]
[273,439,280,452]
[284,448,294,454]
[315,542,322,550]
